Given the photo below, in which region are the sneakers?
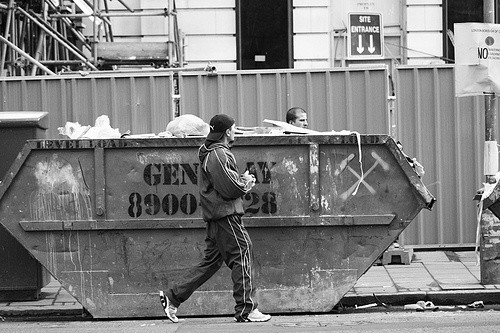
[160,290,179,323]
[234,307,271,322]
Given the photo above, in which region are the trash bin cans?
[0,110,51,301]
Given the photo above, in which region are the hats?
[206,114,235,141]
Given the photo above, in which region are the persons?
[286,107,308,129]
[158,114,272,324]
